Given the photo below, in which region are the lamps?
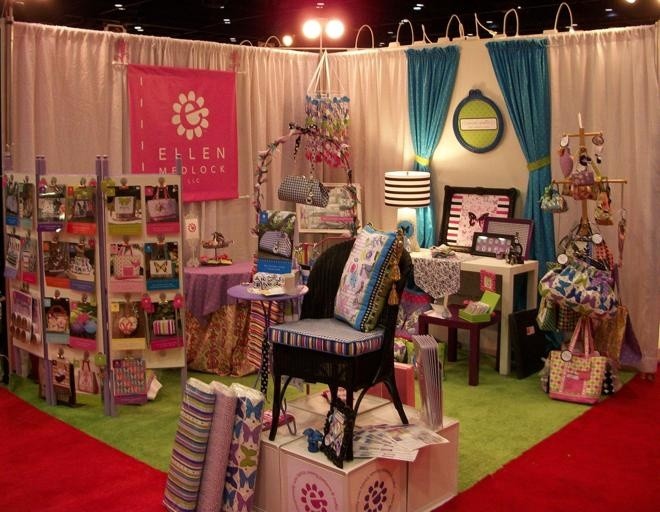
[385,171,429,251]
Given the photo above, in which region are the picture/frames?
[297,183,362,233]
[480,270,496,293]
[484,217,533,261]
[472,231,514,259]
[439,185,520,253]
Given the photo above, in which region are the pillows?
[333,224,404,338]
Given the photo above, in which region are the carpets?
[0,347,638,494]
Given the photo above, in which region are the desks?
[227,284,309,398]
[410,248,538,375]
[184,258,281,375]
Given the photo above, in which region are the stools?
[419,303,501,385]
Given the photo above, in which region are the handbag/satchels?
[106,185,184,351]
[536,234,642,405]
[38,357,147,407]
[278,175,329,207]
[4,182,44,359]
[536,183,567,213]
[37,184,97,351]
[259,231,292,257]
[594,176,615,225]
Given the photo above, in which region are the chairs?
[268,240,414,462]
